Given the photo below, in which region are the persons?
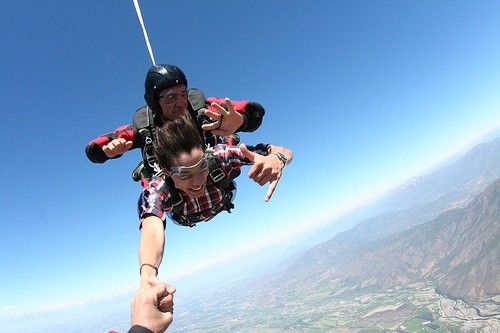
[85,63,265,190]
[136,109,294,313]
[125,284,173,332]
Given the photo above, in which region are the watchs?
[271,150,287,168]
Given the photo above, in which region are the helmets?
[144,63,188,114]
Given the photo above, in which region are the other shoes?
[132,160,144,181]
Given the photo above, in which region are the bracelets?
[140,263,158,277]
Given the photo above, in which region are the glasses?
[170,155,208,180]
[159,88,189,102]
